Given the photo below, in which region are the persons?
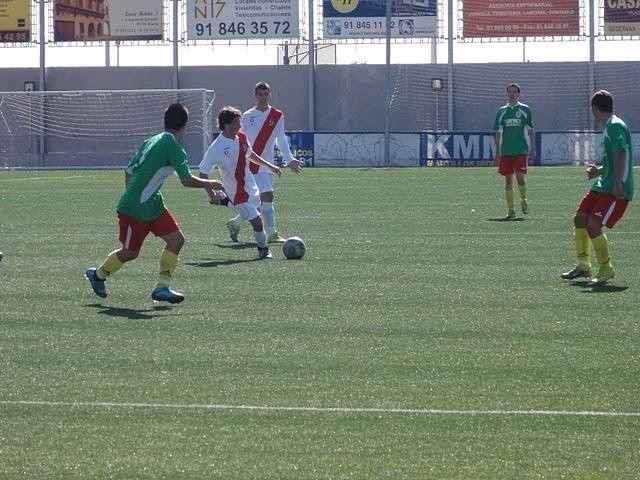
[240,81,305,244]
[199,105,282,259]
[85,104,222,304]
[561,90,635,286]
[492,83,537,219]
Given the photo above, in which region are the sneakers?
[520,201,529,214]
[226,221,240,243]
[506,208,516,219]
[588,265,616,286]
[560,266,591,280]
[85,269,108,298]
[266,233,288,244]
[150,286,184,304]
[256,247,272,259]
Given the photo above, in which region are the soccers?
[283,236,306,260]
